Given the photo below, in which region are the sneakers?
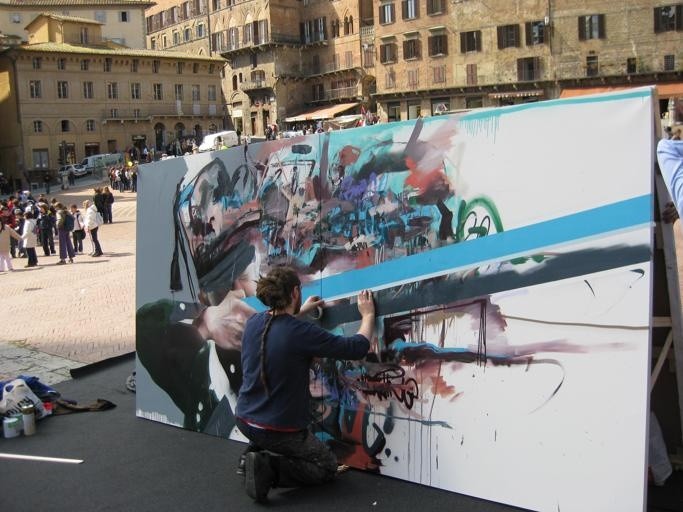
[56,261,66,265]
[245,452,277,505]
[92,251,103,257]
[236,455,246,477]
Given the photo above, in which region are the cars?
[59,164,88,177]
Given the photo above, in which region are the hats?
[195,227,254,289]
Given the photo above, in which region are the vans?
[81,153,111,174]
[198,130,239,152]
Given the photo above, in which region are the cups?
[1,414,23,438]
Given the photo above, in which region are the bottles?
[21,404,35,435]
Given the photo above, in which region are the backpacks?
[60,212,75,231]
[28,219,40,234]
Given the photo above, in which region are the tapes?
[307,305,323,321]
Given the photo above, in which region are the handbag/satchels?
[0,375,61,402]
[80,229,86,239]
[88,207,104,226]
[0,378,53,422]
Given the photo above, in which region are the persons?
[670,129,680,140]
[265,123,283,141]
[236,128,241,145]
[108,142,154,192]
[292,123,324,136]
[165,135,199,157]
[235,265,376,503]
[1,174,115,269]
[663,126,674,140]
[656,137,683,225]
